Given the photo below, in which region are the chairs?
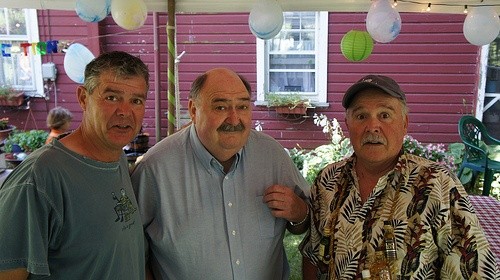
[457,116,500,196]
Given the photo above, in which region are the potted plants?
[265,89,308,116]
[0,86,24,107]
[0,118,16,143]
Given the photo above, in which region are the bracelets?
[289,202,308,227]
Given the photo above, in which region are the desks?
[465,194,500,280]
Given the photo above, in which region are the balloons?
[462,6,500,47]
[64,44,96,84]
[75,0,147,30]
[366,0,402,44]
[248,0,284,40]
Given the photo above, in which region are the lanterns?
[340,30,372,62]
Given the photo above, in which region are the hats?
[342,75,406,110]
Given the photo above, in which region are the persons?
[45,106,74,144]
[0,50,150,280]
[129,67,312,280]
[298,74,500,280]
